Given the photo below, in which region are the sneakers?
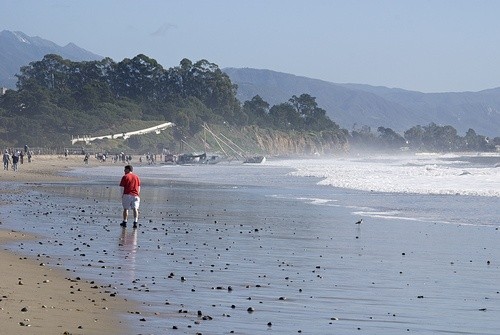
[133,222,137,228]
[120,221,127,227]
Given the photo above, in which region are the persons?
[118,228,138,261]
[0,144,156,172]
[118,164,141,228]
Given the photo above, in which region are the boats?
[241,155,267,163]
[168,152,223,164]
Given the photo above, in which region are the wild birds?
[355,219,363,225]
